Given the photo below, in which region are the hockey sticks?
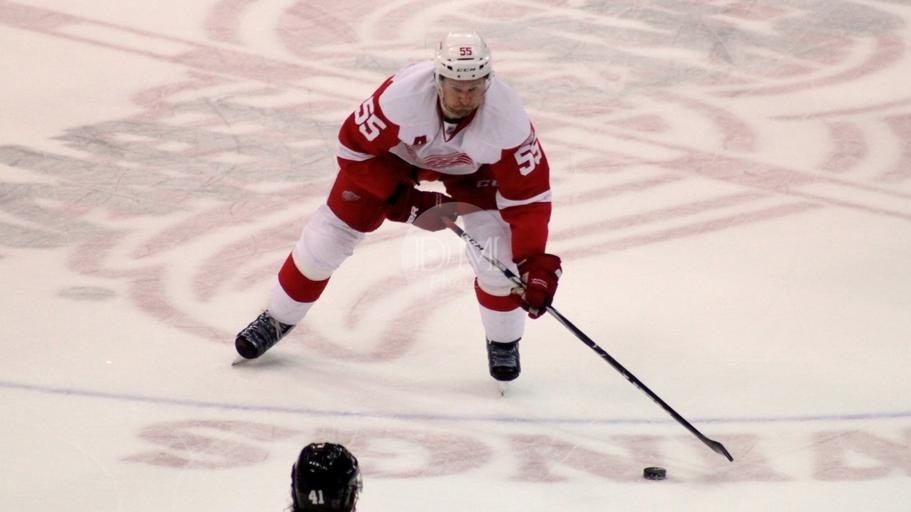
[443,218,733,462]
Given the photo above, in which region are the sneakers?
[234,308,298,361]
[485,335,522,383]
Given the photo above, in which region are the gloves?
[381,182,459,233]
[509,252,564,320]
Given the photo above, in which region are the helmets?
[433,31,494,81]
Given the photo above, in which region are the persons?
[234,25,563,383]
